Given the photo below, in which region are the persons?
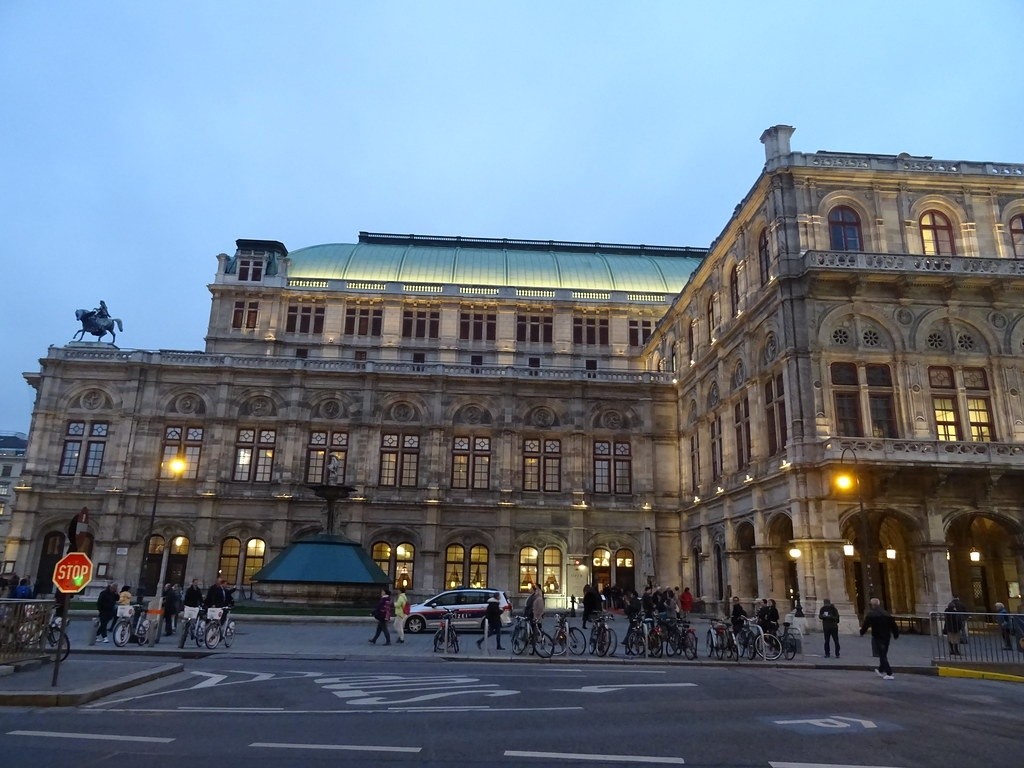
[819,598,899,680]
[476,593,506,650]
[326,456,338,477]
[184,578,204,619]
[643,587,655,629]
[204,576,237,625]
[679,587,693,621]
[162,583,184,636]
[528,584,545,645]
[757,599,780,651]
[943,595,1012,655]
[652,586,663,612]
[92,300,111,318]
[660,586,676,642]
[0,570,35,648]
[368,586,392,646]
[602,583,624,610]
[731,596,747,637]
[582,582,604,630]
[518,585,536,641]
[673,587,681,619]
[96,582,120,642]
[621,591,641,645]
[393,586,408,643]
[53,588,74,617]
[107,585,132,633]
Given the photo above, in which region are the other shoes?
[382,642,392,645]
[875,668,883,677]
[83,623,233,642]
[497,645,506,650]
[835,654,840,658]
[824,654,831,658]
[396,638,405,643]
[883,673,895,680]
[476,640,482,649]
[368,638,376,644]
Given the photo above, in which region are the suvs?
[402,587,514,634]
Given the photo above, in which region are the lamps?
[885,518,896,558]
[966,525,979,561]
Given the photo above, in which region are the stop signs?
[52,553,93,594]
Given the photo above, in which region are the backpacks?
[403,601,411,615]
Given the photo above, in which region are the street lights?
[837,448,869,622]
[133,430,186,630]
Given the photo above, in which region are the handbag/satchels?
[374,610,386,620]
[960,632,969,645]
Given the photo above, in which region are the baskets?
[208,608,224,619]
[185,607,199,619]
[117,606,134,617]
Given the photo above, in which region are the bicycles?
[112,604,152,647]
[18,604,70,662]
[700,614,797,661]
[625,611,698,660]
[511,615,586,658]
[433,607,459,653]
[178,605,236,649]
[588,611,618,657]
